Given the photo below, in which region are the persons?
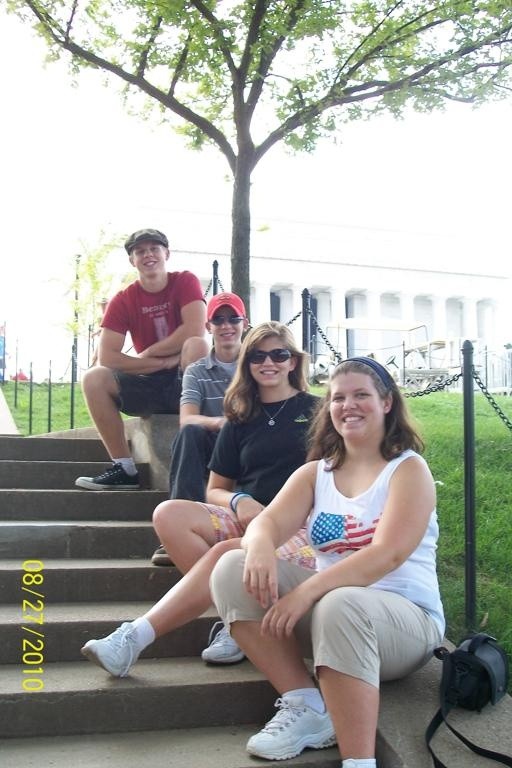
[81,321,328,680]
[208,357,447,767]
[75,227,209,491]
[152,291,245,563]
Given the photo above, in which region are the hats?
[124,228,168,254]
[207,292,246,320]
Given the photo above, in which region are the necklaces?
[255,388,294,426]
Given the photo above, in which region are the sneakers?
[74,461,140,491]
[202,621,244,663]
[245,678,338,761]
[151,544,171,564]
[80,622,142,678]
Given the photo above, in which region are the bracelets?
[229,492,252,513]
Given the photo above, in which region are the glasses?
[208,315,245,325]
[249,348,292,364]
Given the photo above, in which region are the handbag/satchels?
[425,632,512,768]
